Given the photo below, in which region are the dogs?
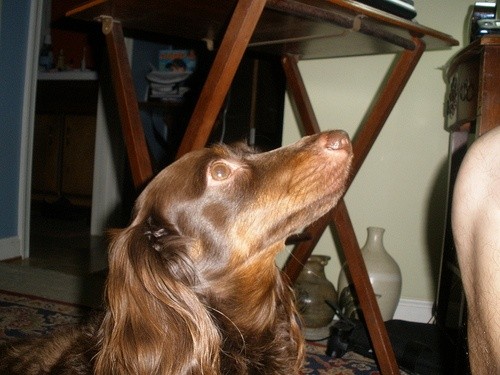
[0,127,353,375]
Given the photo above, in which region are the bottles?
[56,48,65,68]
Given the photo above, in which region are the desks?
[63,0,460,375]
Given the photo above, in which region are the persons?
[451,123,500,375]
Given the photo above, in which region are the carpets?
[0,289,414,375]
